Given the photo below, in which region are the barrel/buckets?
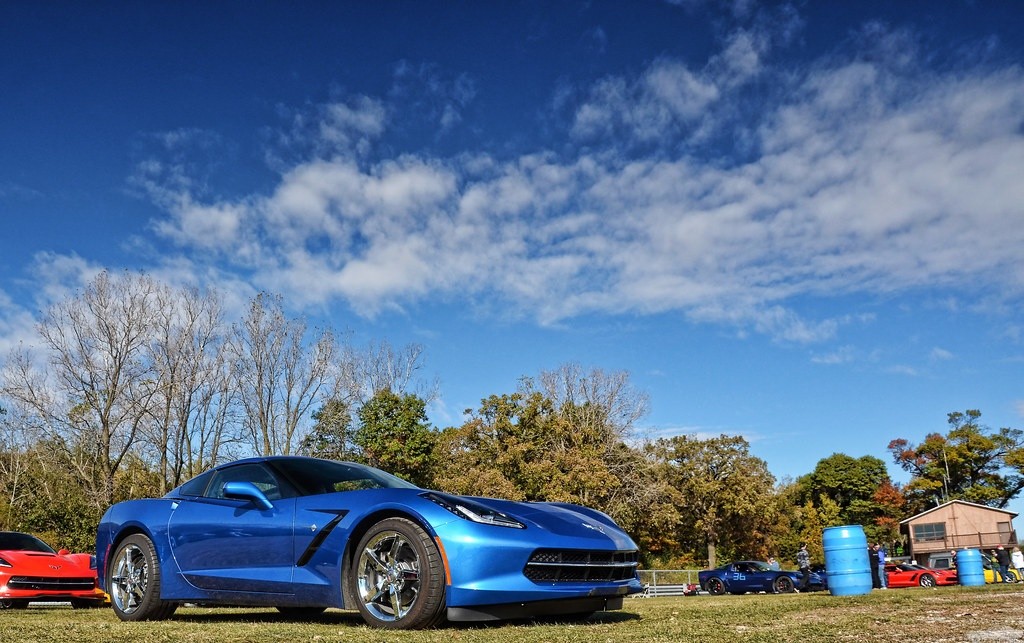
[956,548,985,586]
[822,525,873,596]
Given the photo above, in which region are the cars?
[951,553,1021,584]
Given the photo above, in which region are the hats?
[874,543,878,546]
[996,545,1003,548]
[991,550,995,554]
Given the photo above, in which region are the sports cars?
[883,563,958,588]
[698,561,828,596]
[0,456,644,630]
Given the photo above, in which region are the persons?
[868,543,879,589]
[874,544,887,589]
[793,542,809,593]
[769,558,779,569]
[950,550,960,586]
[993,545,1014,583]
[1012,547,1024,583]
[990,550,1001,583]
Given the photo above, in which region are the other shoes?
[1018,581,1023,583]
[794,588,799,593]
[880,587,887,590]
[872,587,879,590]
